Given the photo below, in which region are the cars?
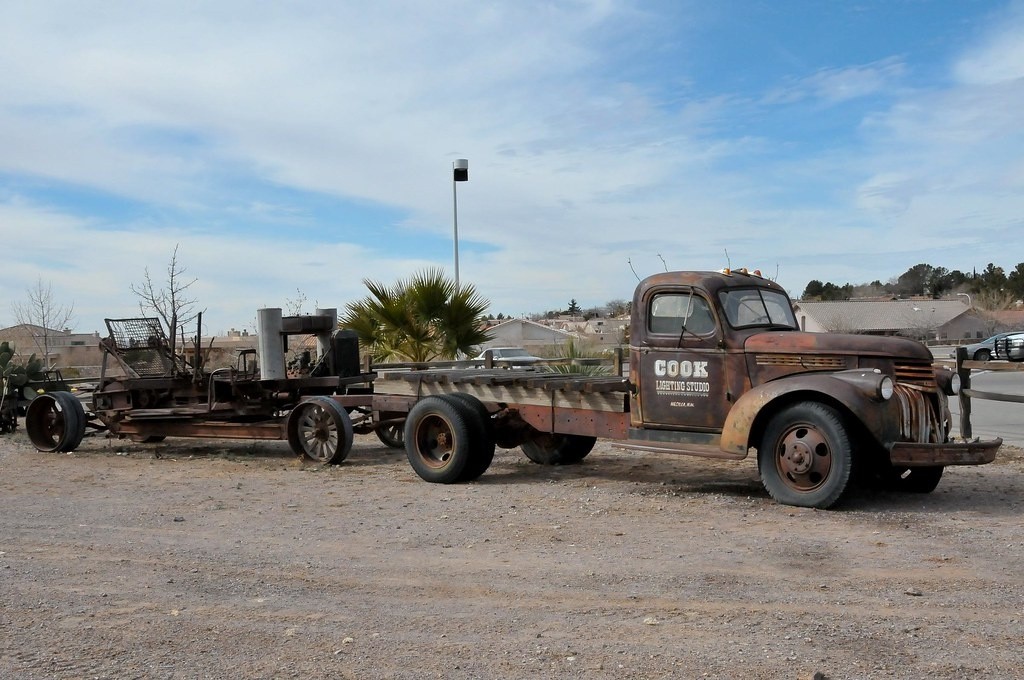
[948,331,1004,362]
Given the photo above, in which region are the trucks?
[372,260,1002,511]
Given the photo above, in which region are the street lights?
[453,158,469,294]
[956,292,971,307]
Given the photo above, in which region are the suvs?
[989,331,1024,362]
